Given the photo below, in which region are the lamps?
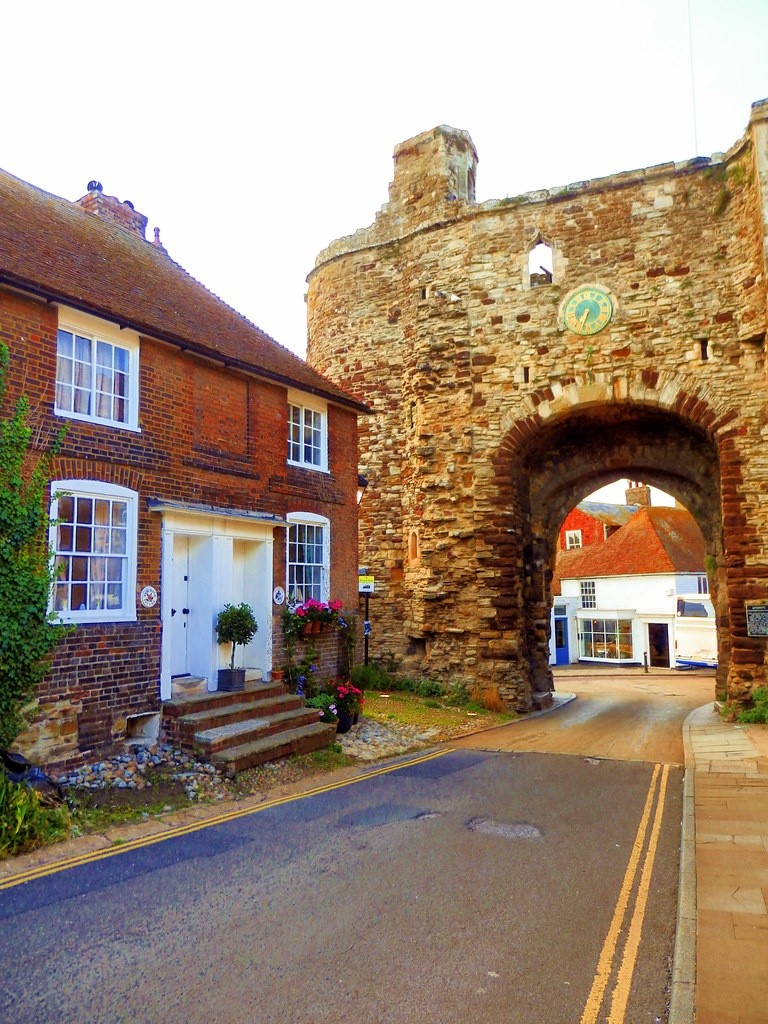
[357,475,369,504]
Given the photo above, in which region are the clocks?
[563,288,612,336]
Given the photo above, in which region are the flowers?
[295,599,343,626]
[291,664,366,723]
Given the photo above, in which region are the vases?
[302,620,321,634]
[332,711,359,733]
[304,679,312,686]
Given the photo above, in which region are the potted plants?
[271,666,284,682]
[215,602,258,691]
